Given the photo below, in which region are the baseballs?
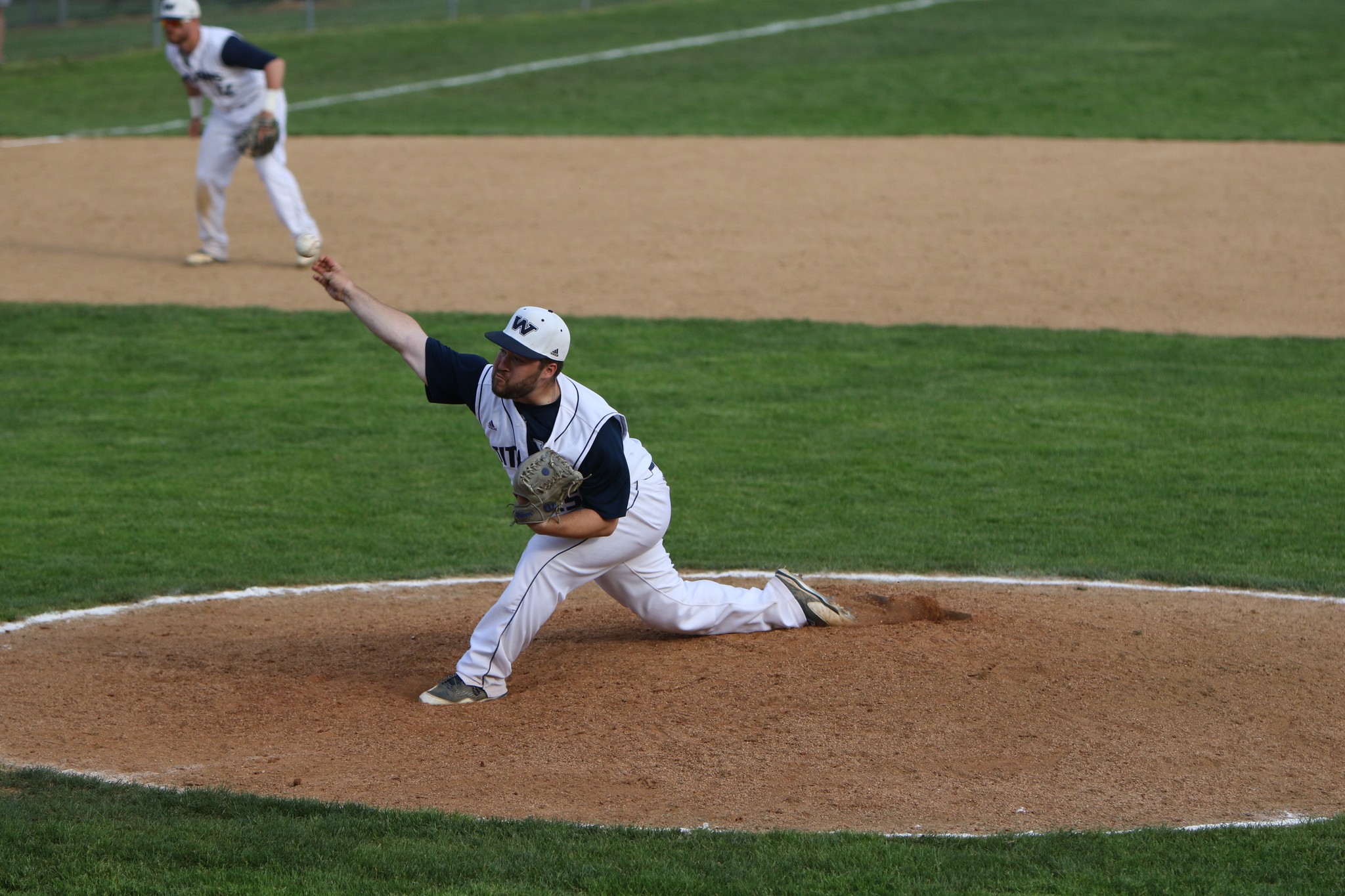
[295,232,322,258]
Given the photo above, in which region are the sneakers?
[418,673,508,707]
[184,251,212,265]
[297,255,315,265]
[776,568,856,628]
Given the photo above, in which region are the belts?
[647,461,656,471]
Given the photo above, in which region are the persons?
[153,0,319,268]
[312,256,855,704]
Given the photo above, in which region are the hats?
[485,306,571,362]
[154,2,202,22]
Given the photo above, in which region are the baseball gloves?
[513,447,583,525]
[234,112,280,161]
